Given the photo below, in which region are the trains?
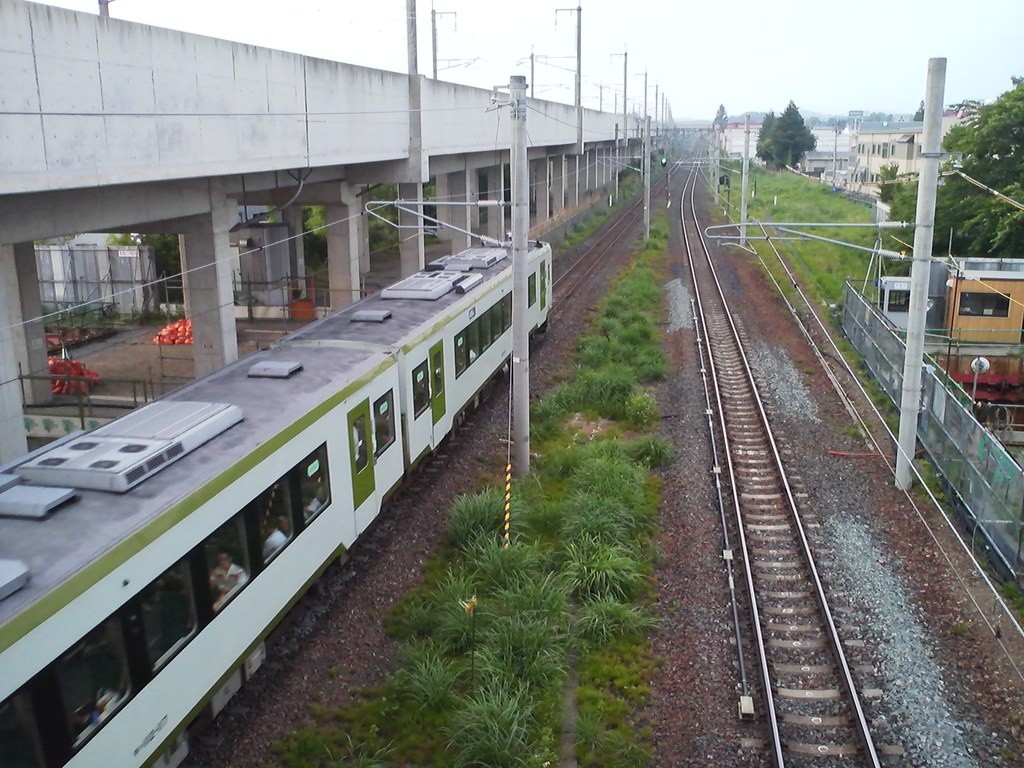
[0,235,553,768]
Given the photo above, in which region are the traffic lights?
[659,157,667,165]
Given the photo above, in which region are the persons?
[302,491,321,520]
[263,518,288,560]
[210,547,247,602]
[352,425,360,473]
